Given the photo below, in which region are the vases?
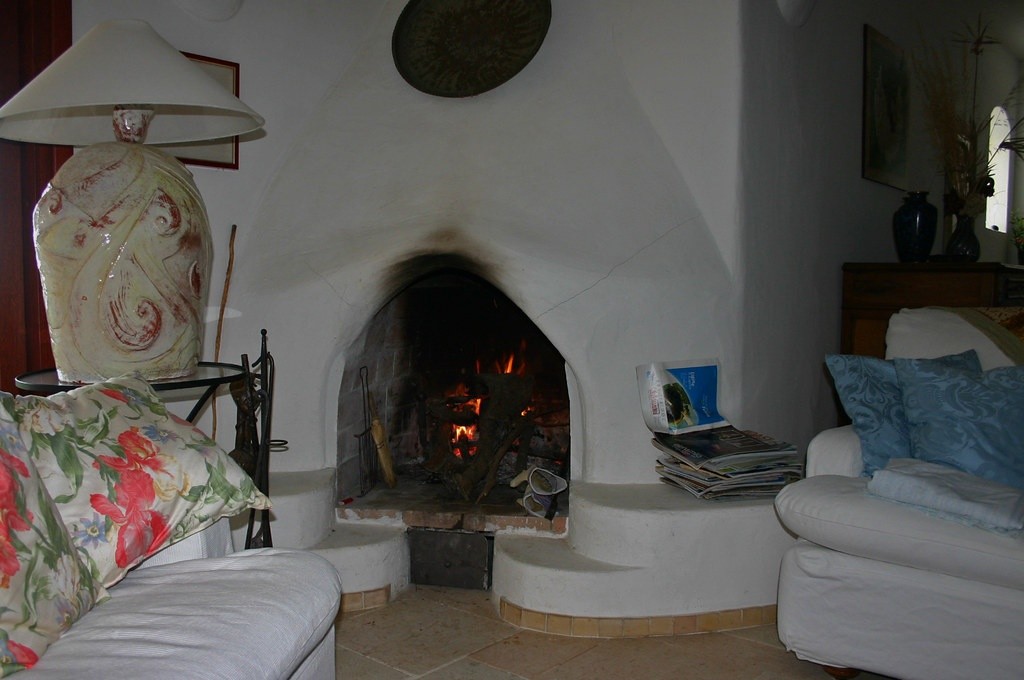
[893,190,938,262]
[1018,248,1024,265]
[945,214,981,261]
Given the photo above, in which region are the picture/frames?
[176,51,241,170]
[862,24,910,190]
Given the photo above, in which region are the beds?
[775,305,1024,680]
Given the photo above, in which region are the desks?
[836,261,1024,427]
[14,330,275,552]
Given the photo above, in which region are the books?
[649,423,803,503]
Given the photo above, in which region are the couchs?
[0,514,343,680]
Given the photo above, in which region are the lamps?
[0,18,267,384]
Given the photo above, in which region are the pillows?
[0,400,112,679]
[825,350,982,477]
[0,369,273,590]
[893,355,1024,487]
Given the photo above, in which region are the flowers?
[908,8,1024,219]
[1006,208,1024,251]
